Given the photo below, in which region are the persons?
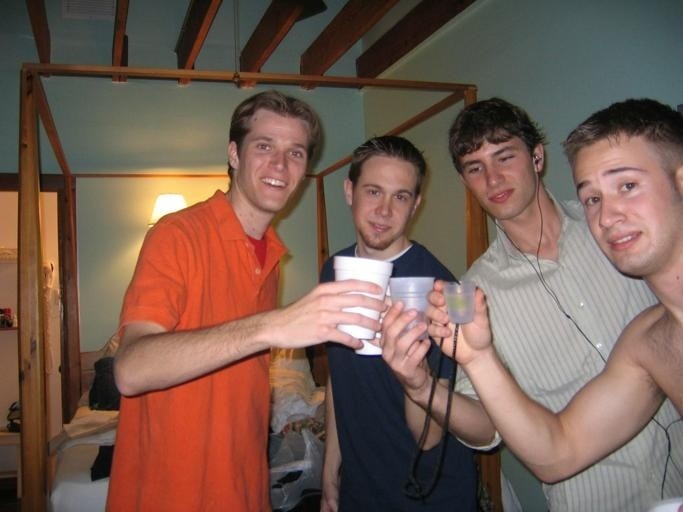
[377,93,683,512]
[317,131,488,512]
[105,86,393,512]
[421,98,683,485]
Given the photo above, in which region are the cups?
[332,255,480,358]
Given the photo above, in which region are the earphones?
[534,155,540,164]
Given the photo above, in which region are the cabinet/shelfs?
[0,246,63,479]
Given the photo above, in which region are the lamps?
[147,193,187,228]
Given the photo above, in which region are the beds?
[19,62,502,512]
